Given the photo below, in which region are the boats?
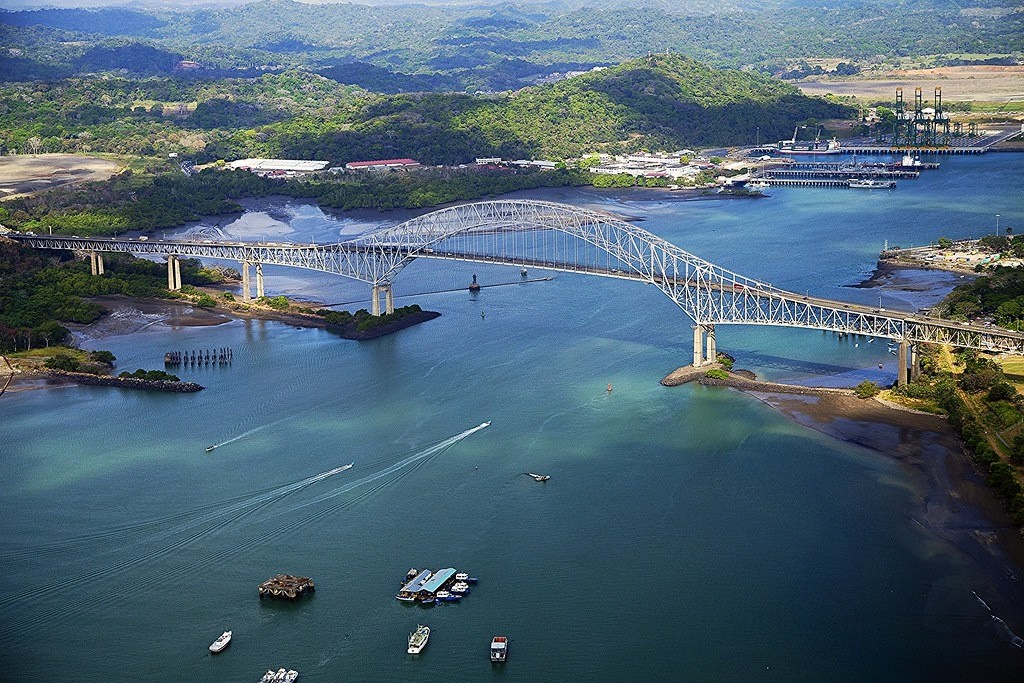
[749,183,769,190]
[206,445,213,451]
[534,475,550,482]
[407,627,430,654]
[849,179,895,189]
[346,461,354,468]
[490,636,508,663]
[396,566,478,607]
[207,632,233,653]
[480,420,491,430]
[258,668,299,683]
